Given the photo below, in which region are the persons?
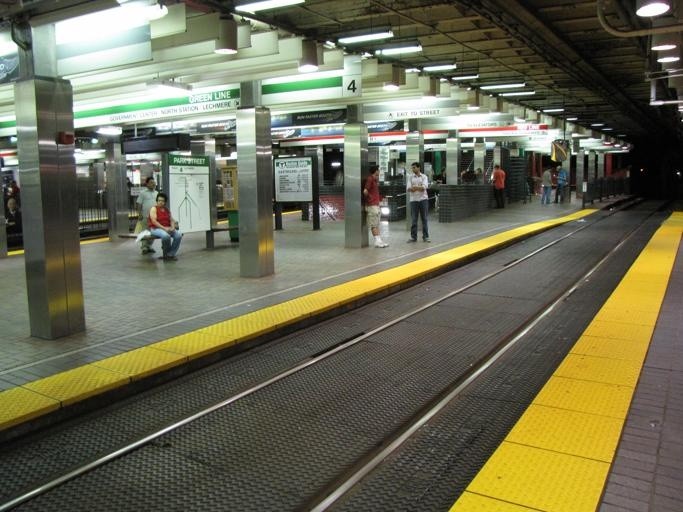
[489,165,506,209]
[362,165,390,248]
[135,176,160,255]
[551,165,567,205]
[125,177,134,217]
[147,194,184,262]
[406,162,432,243]
[540,165,555,204]
[461,167,482,184]
[5,180,21,197]
[5,197,22,252]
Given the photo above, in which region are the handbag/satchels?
[134,215,143,234]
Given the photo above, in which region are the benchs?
[118,229,183,254]
[206,227,239,248]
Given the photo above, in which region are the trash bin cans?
[228,209,240,243]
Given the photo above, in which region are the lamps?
[634,0,683,66]
[338,10,567,121]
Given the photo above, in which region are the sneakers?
[409,238,416,241]
[374,243,388,248]
[143,249,177,261]
[424,238,431,242]
